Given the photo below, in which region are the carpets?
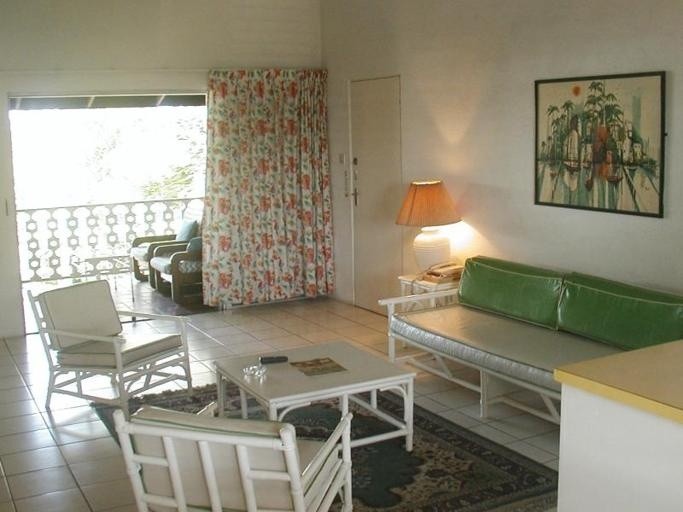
[89,379,558,512]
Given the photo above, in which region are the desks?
[553,342,683,511]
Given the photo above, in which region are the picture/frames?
[534,71,666,219]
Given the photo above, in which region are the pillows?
[174,221,199,242]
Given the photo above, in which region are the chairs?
[111,403,352,510]
[132,235,203,305]
[27,281,194,422]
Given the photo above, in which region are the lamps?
[394,180,463,273]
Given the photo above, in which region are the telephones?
[429,262,464,276]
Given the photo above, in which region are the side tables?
[398,276,460,349]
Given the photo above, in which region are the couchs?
[378,254,683,419]
